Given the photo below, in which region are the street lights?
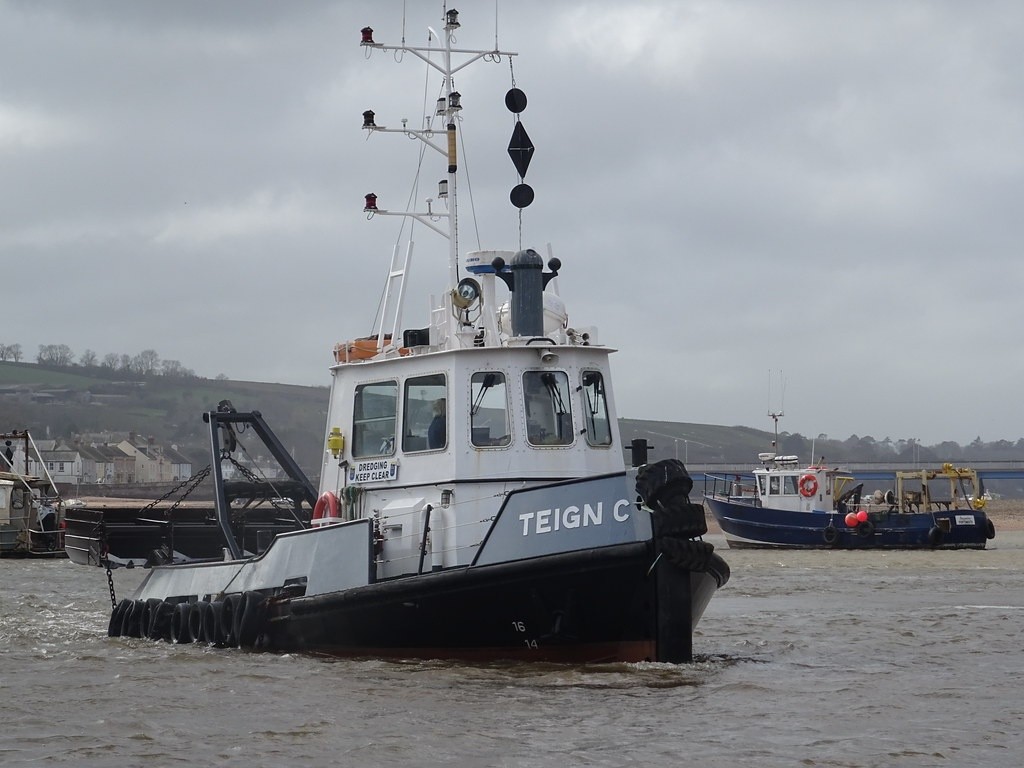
[675,440,677,459]
[685,440,687,466]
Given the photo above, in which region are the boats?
[0,429,68,557]
[64,1,729,663]
[701,369,986,549]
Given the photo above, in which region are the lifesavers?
[105,589,266,650]
[308,492,338,528]
[822,525,840,545]
[928,527,946,546]
[984,516,996,541]
[856,520,875,539]
[797,473,819,498]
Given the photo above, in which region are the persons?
[733,476,742,496]
[428,397,446,449]
